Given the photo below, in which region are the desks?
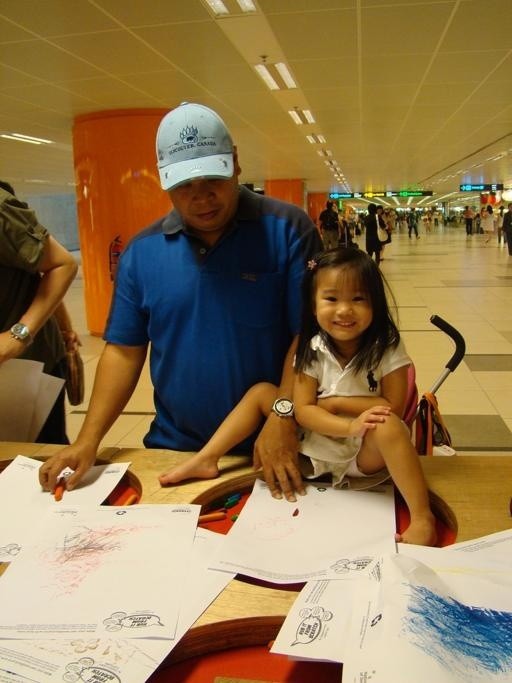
[1,442,124,514]
[1,447,512,683]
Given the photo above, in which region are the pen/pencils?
[225,498,238,507]
[55,477,66,500]
[212,498,227,507]
[123,495,137,506]
[225,492,242,501]
[231,511,241,520]
[198,512,225,522]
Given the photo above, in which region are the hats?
[155,100,237,193]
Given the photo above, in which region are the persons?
[404,204,462,239]
[0,186,78,447]
[364,204,404,261]
[319,200,364,249]
[157,244,436,548]
[39,102,326,500]
[462,202,512,256]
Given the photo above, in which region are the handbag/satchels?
[64,348,86,406]
[375,212,389,243]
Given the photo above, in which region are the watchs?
[10,323,34,347]
[271,397,294,418]
